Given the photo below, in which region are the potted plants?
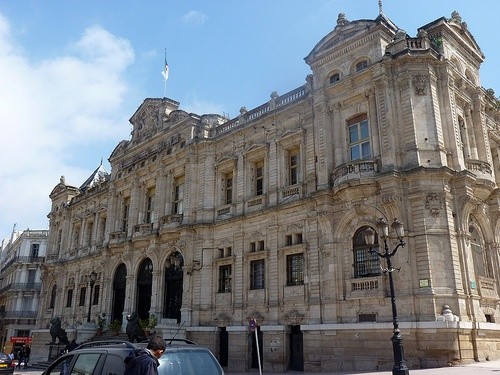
[96,317,106,336]
[110,319,121,336]
[141,315,157,336]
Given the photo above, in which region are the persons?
[8,345,32,370]
[125,335,166,375]
[62,339,79,363]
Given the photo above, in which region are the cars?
[0,352,15,375]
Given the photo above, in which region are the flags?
[162,51,168,80]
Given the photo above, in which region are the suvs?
[41,338,225,375]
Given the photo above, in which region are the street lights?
[364,217,409,375]
[86,271,98,323]
